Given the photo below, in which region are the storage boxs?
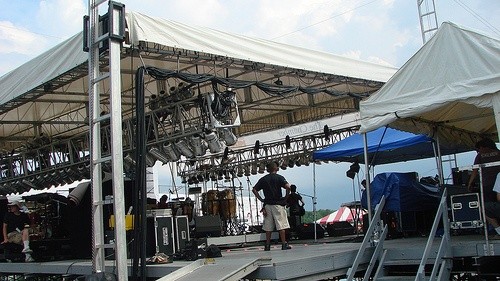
[147,209,190,257]
[450,193,483,229]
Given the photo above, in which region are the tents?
[0,9,400,281]
[358,18,500,247]
[313,126,442,243]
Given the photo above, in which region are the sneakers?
[22,248,35,254]
[22,256,34,263]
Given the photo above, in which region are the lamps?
[0,82,331,197]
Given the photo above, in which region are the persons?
[468,138,500,240]
[286,184,305,239]
[252,162,293,251]
[361,179,366,191]
[1,200,36,262]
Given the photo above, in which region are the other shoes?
[282,243,293,249]
[264,243,270,250]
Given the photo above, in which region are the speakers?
[193,216,221,237]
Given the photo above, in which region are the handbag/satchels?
[297,205,305,216]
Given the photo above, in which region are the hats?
[8,200,21,208]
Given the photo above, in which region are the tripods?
[224,172,259,236]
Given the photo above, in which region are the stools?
[0,242,25,263]
